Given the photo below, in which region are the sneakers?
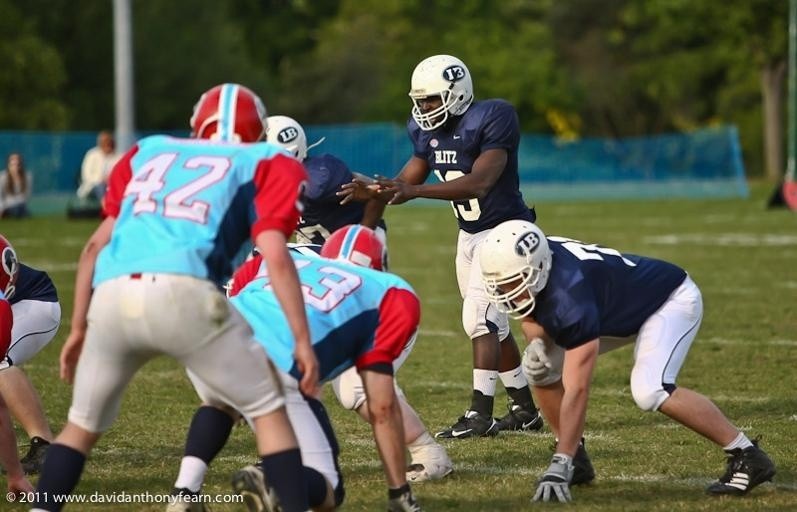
[534,441,594,488]
[434,405,544,440]
[1,437,50,481]
[405,465,454,485]
[232,463,281,512]
[707,440,776,497]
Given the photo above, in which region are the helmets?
[409,54,475,130]
[189,82,307,162]
[481,220,553,322]
[320,224,387,271]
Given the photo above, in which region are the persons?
[0,234,36,501]
[0,154,33,218]
[266,114,454,483]
[164,222,423,512]
[77,130,123,209]
[0,263,62,476]
[335,54,544,439]
[24,82,321,512]
[479,218,777,504]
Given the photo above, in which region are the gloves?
[531,454,575,503]
[522,339,552,388]
[387,484,421,512]
